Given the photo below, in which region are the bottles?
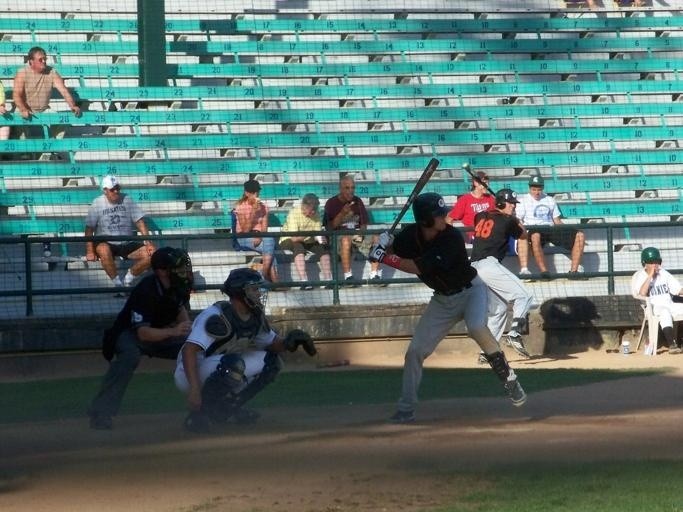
[622,341,629,354]
[43,241,51,257]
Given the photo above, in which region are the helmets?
[496,189,520,209]
[153,248,194,299]
[225,268,275,320]
[413,192,454,228]
[642,246,661,262]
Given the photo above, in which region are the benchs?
[0,2,683,256]
[537,289,681,357]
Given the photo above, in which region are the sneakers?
[90,412,113,431]
[668,339,682,353]
[506,380,526,408]
[224,407,260,426]
[185,413,213,434]
[506,335,532,358]
[389,410,415,424]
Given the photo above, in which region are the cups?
[316,235,323,245]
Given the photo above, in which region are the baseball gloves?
[285,329,317,356]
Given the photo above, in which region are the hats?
[529,176,544,185]
[102,174,120,189]
[244,180,263,192]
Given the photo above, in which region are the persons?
[0,79,12,140]
[172,268,317,432]
[323,175,381,288]
[564,0,600,8]
[632,247,683,354]
[615,0,650,7]
[277,193,335,289]
[370,193,527,424]
[446,171,536,281]
[515,176,585,281]
[469,188,534,364]
[235,180,287,291]
[12,47,81,159]
[87,246,195,431]
[85,176,156,297]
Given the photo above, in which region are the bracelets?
[647,277,653,283]
[383,255,400,270]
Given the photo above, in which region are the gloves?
[368,244,387,263]
[379,231,394,250]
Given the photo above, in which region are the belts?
[442,283,472,296]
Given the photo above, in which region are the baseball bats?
[370,159,439,262]
[462,162,495,197]
